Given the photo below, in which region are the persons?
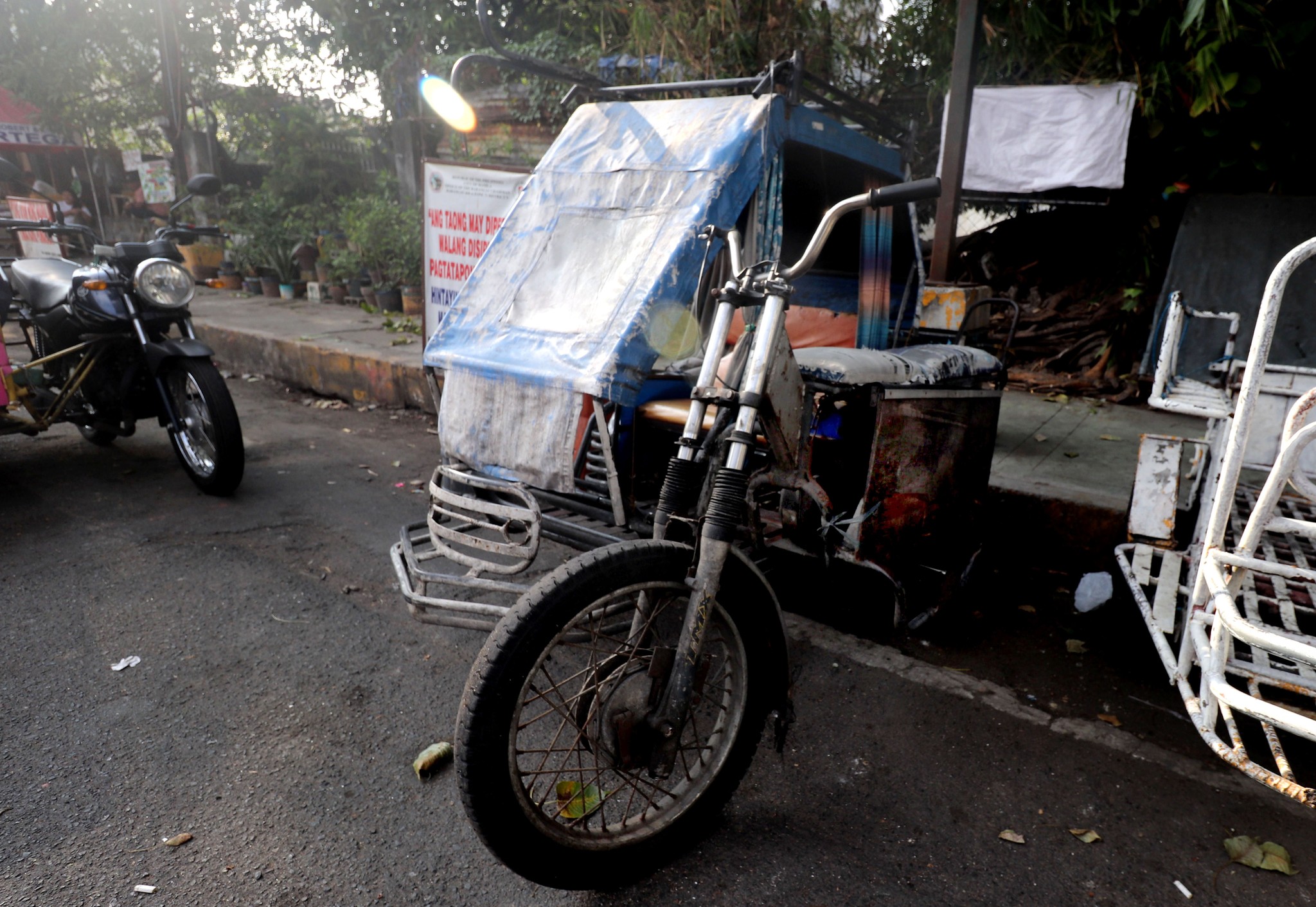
[23,171,62,202]
[53,190,92,258]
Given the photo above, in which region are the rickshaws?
[418,45,1009,892]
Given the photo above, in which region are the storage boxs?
[917,285,992,335]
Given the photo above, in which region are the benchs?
[637,296,862,454]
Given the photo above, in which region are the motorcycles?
[0,157,246,496]
